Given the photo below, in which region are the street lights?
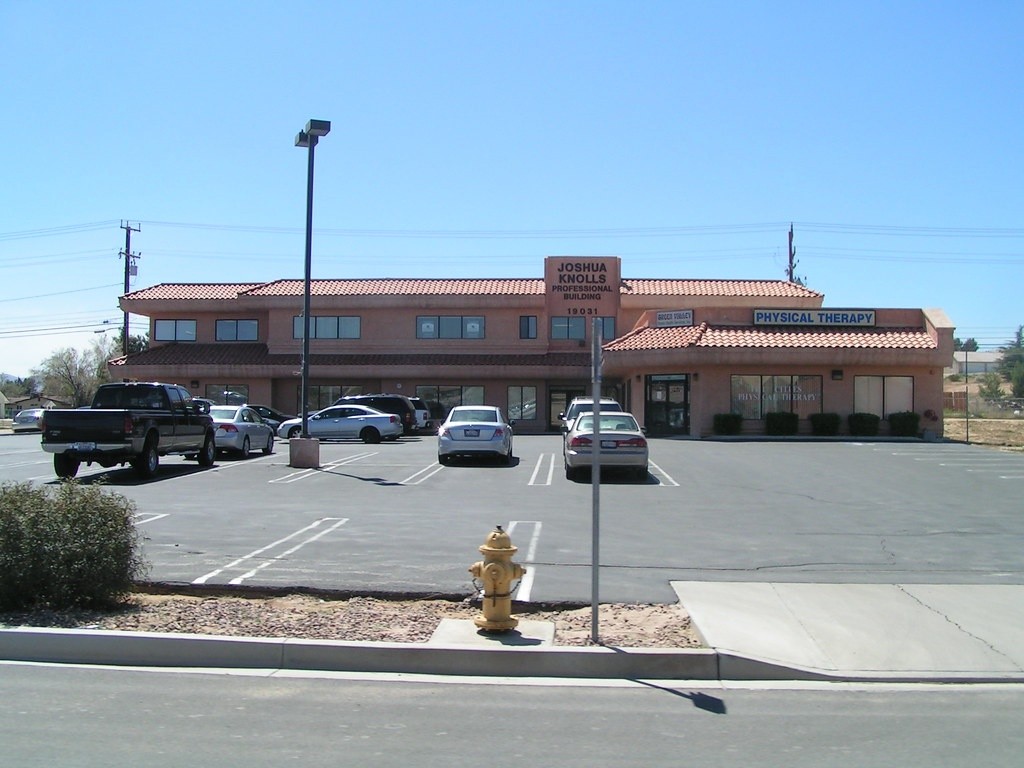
[289,119,331,469]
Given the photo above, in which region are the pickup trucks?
[41,381,216,481]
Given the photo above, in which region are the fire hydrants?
[469,524,527,633]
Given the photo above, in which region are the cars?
[243,404,299,423]
[402,396,431,436]
[277,405,405,445]
[12,409,46,433]
[438,405,515,464]
[184,405,275,460]
[559,411,648,479]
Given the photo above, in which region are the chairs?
[583,423,595,428]
[616,424,626,430]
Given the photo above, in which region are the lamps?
[831,369,843,380]
[191,380,199,388]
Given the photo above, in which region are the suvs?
[557,396,624,456]
[297,394,416,441]
